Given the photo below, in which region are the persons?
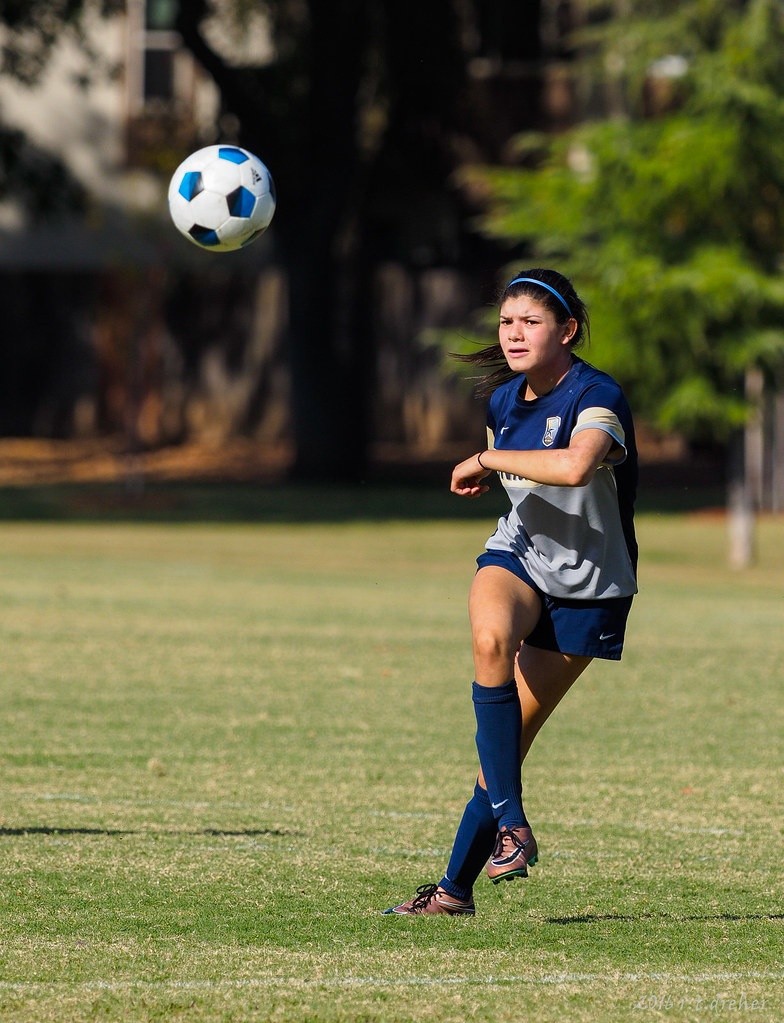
[381,269,640,916]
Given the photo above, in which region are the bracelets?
[478,448,489,471]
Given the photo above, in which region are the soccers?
[167,143,276,253]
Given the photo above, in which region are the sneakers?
[486,826,540,885]
[382,887,475,918]
